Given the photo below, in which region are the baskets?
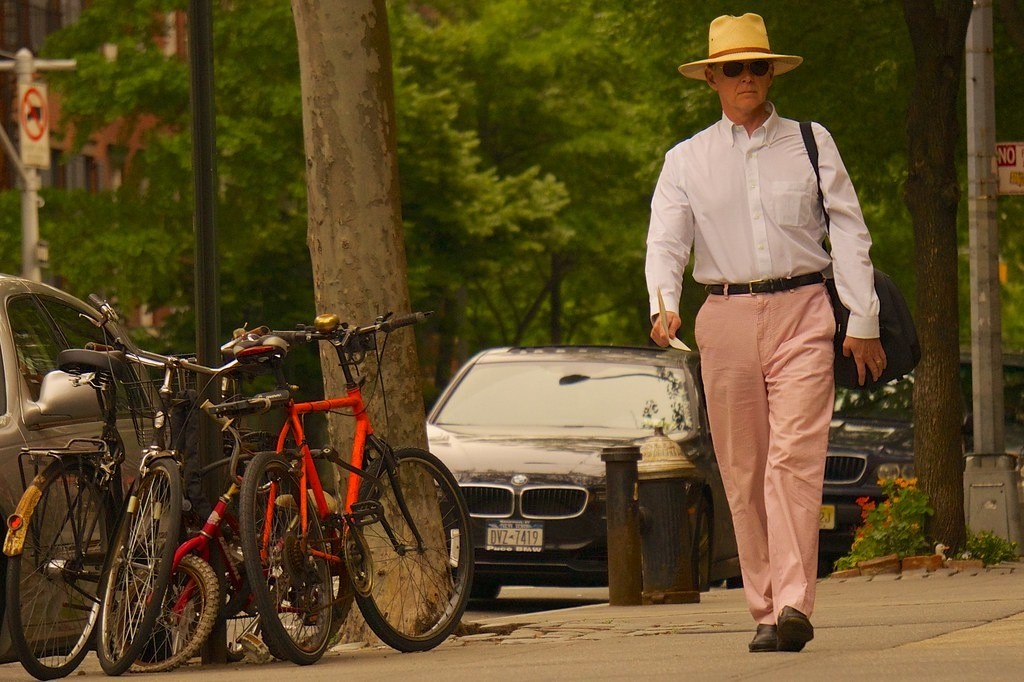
[117,353,245,450]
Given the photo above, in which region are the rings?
[876,360,881,363]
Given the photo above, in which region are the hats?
[677,13,803,81]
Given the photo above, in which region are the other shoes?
[777,604,814,651]
[748,623,776,651]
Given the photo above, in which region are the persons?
[644,12,888,653]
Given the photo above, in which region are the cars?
[0,272,174,663]
[424,342,742,601]
[816,352,1024,580]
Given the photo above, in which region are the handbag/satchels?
[827,268,922,389]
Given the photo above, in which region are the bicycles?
[1,292,474,680]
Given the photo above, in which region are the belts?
[707,272,824,295]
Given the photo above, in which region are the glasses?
[711,59,772,77]
